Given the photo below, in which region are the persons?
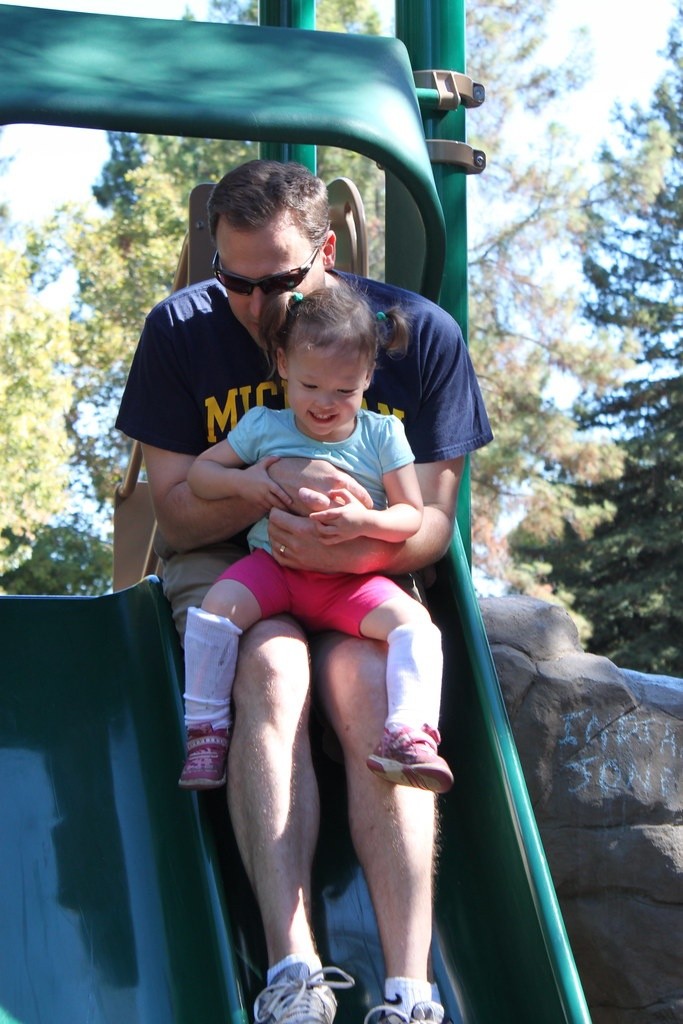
[177,287,462,795]
[112,160,496,1023]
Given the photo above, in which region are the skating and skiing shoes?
[363,1001,445,1024]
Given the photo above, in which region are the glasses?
[212,248,320,297]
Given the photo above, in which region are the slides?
[0,575,594,1024]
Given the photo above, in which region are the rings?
[279,544,287,557]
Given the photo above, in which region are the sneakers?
[253,962,354,1023]
[177,725,230,788]
[366,722,453,795]
